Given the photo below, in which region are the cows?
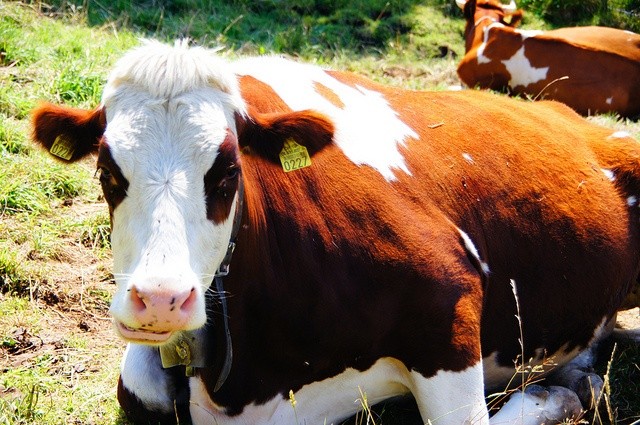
[28,37,640,425]
[456,0,640,123]
